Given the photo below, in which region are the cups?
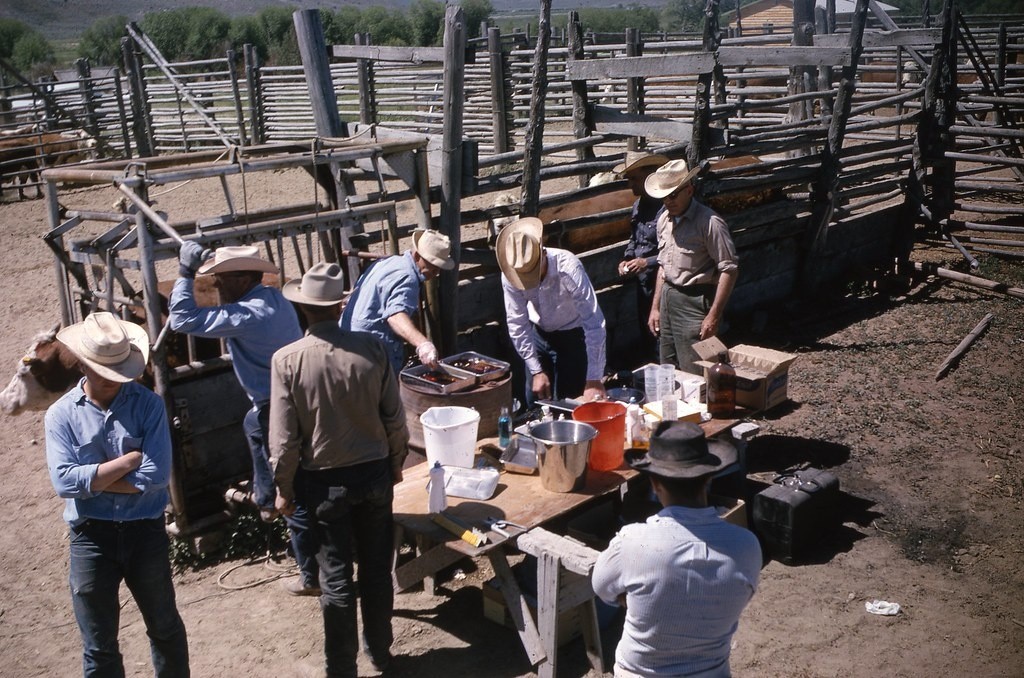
[643,363,678,432]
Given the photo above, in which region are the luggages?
[752,467,840,566]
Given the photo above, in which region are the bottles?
[631,408,649,448]
[626,396,640,445]
[705,351,736,419]
[498,407,512,447]
[526,406,566,434]
[428,460,448,513]
[591,395,605,402]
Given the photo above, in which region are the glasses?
[623,174,642,179]
[211,273,234,280]
[663,186,689,199]
[422,258,439,279]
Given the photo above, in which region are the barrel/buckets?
[531,420,601,492]
[572,401,627,471]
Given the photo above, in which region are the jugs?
[419,405,480,470]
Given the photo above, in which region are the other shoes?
[370,653,389,672]
[285,579,322,596]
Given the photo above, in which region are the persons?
[168,238,320,599]
[270,260,408,678]
[42,310,192,678]
[612,151,740,374]
[336,230,456,370]
[497,215,608,401]
[590,421,765,676]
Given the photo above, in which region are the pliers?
[484,516,527,539]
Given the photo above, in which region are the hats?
[496,216,543,291]
[623,420,738,479]
[282,261,353,306]
[56,312,149,382]
[198,247,279,274]
[613,151,669,173]
[412,228,454,270]
[644,158,701,198]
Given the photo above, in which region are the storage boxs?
[400,362,477,393]
[691,334,798,410]
[438,350,511,383]
[705,493,748,530]
[483,527,623,649]
[751,466,839,556]
[499,436,539,474]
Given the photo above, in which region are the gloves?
[180,240,211,271]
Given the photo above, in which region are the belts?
[87,520,152,530]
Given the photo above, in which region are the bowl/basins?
[608,387,646,405]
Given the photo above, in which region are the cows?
[0,125,98,199]
[483,150,772,254]
[0,272,298,416]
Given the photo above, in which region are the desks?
[390,405,758,678]
